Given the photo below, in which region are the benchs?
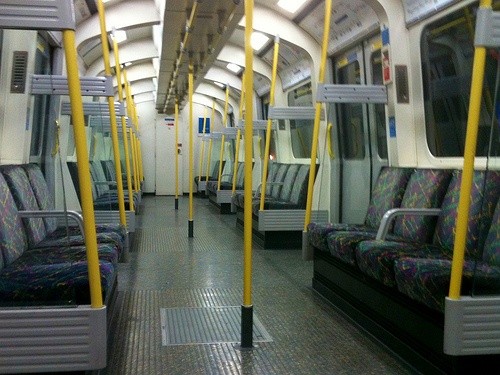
[229,163,319,249]
[0,162,127,373]
[194,159,226,199]
[114,159,145,181]
[66,160,138,213]
[101,161,143,190]
[206,161,255,213]
[306,165,500,374]
